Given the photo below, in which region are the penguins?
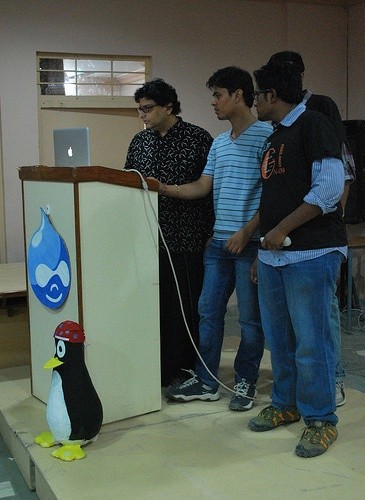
[33,319,104,461]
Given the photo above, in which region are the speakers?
[341,119,365,226]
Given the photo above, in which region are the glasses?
[252,90,273,100]
[136,103,165,114]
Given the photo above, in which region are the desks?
[338,237,365,333]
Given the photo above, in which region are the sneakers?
[248,404,301,431]
[165,369,196,399]
[336,380,346,407]
[296,419,339,458]
[169,375,220,403]
[229,378,257,411]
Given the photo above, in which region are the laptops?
[53,127,90,167]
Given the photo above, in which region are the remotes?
[260,236,291,247]
